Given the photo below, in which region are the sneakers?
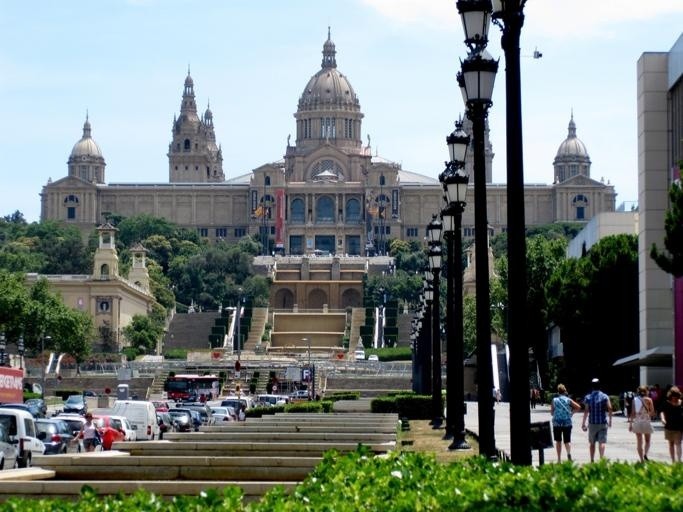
[643,453,649,461]
[566,453,573,462]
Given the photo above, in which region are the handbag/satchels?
[93,427,102,447]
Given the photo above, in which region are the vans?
[354,351,365,360]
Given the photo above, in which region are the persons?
[69,414,102,452]
[582,379,612,463]
[659,386,683,462]
[493,386,502,403]
[550,384,580,463]
[530,386,545,409]
[629,386,655,462]
[619,384,673,423]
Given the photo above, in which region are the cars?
[369,355,379,362]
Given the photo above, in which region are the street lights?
[236,287,244,364]
[300,339,311,365]
[41,334,51,396]
